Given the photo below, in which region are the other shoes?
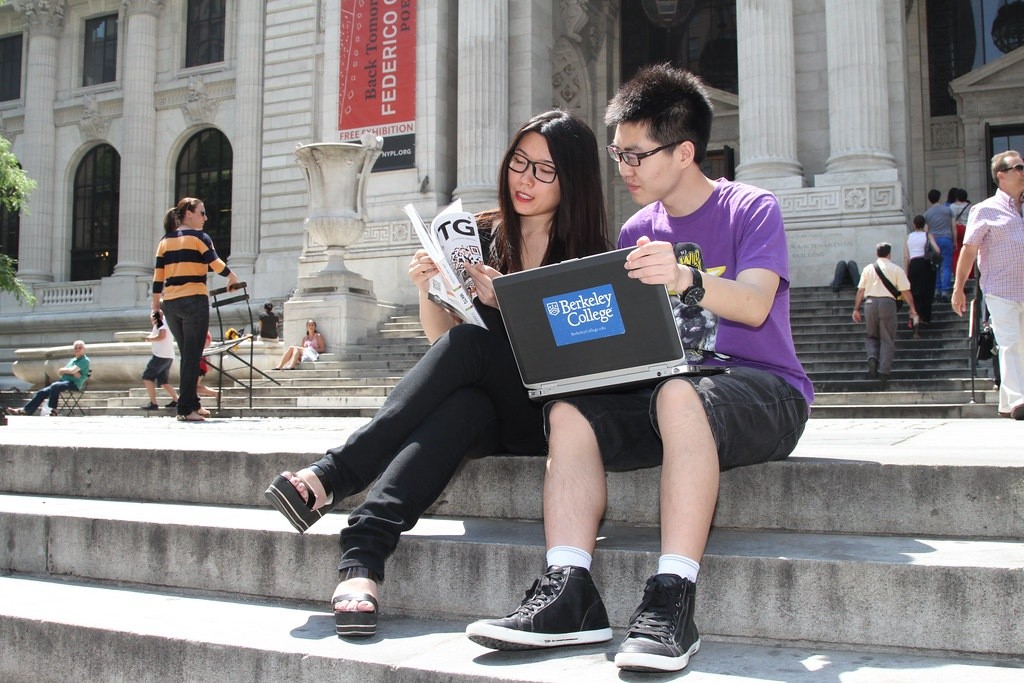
[165,401,179,407]
[271,366,280,370]
[7,407,25,416]
[1011,404,1024,419]
[46,409,58,417]
[283,366,295,371]
[867,359,879,379]
[141,402,158,409]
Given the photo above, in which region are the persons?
[466,60,815,671]
[150,197,239,421]
[257,303,279,342]
[264,102,615,634]
[6,340,90,416]
[921,189,957,303]
[138,298,179,410]
[951,149,1024,419]
[852,241,917,381]
[983,315,1000,390]
[944,187,959,207]
[904,214,940,326]
[950,188,975,280]
[271,319,325,370]
[196,329,223,405]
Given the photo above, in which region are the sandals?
[177,413,205,421]
[193,407,210,415]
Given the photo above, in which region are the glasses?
[605,140,682,167]
[193,209,207,217]
[505,150,559,183]
[999,164,1024,174]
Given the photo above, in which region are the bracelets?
[853,309,860,310]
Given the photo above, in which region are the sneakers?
[466,565,613,650]
[615,574,700,671]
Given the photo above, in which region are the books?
[401,197,489,330]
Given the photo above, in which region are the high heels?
[332,566,377,636]
[264,470,337,533]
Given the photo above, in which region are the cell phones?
[152,311,164,329]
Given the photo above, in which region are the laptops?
[491,245,727,404]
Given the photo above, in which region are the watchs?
[677,266,705,306]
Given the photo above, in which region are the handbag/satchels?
[923,233,942,268]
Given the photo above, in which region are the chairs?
[202,281,283,410]
[58,369,92,417]
